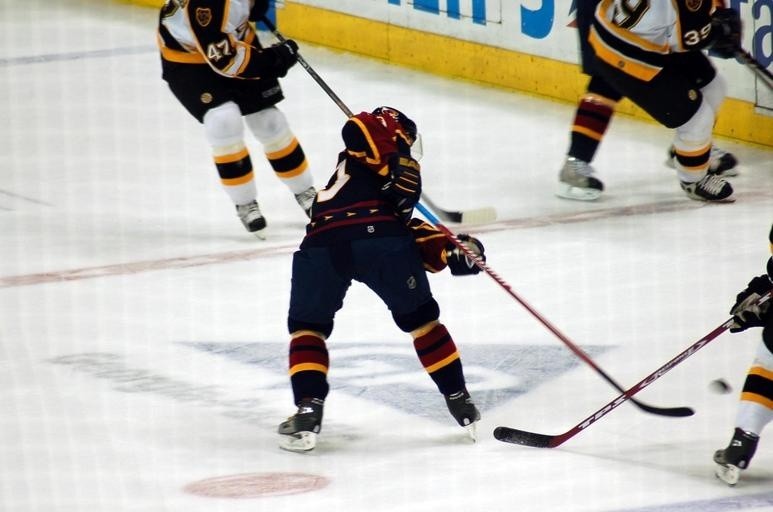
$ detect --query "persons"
[275,105,487,437]
[158,0,320,233]
[558,1,743,200]
[711,222,773,471]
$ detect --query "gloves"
[445,233,488,277]
[380,154,425,218]
[705,6,744,61]
[239,39,299,84]
[729,274,773,334]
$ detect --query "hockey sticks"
[415,205,695,417]
[494,293,770,448]
[265,18,496,225]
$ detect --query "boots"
[275,397,325,437]
[558,152,606,193]
[442,386,484,428]
[713,426,761,470]
[294,185,318,220]
[233,199,270,233]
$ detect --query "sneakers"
[678,173,736,201]
[666,143,741,174]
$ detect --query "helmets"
[370,105,419,153]
